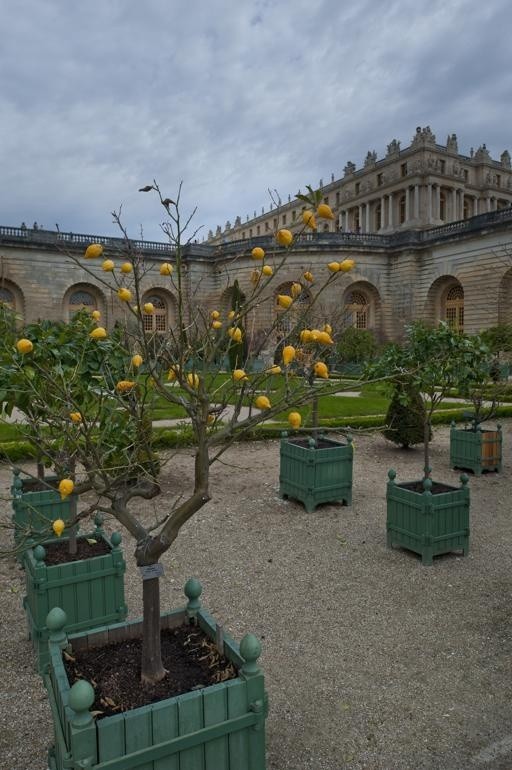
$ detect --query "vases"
[37,577,267,770]
[279,430,354,513]
[12,474,79,546]
[21,513,128,675]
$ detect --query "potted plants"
[363,316,512,566]
